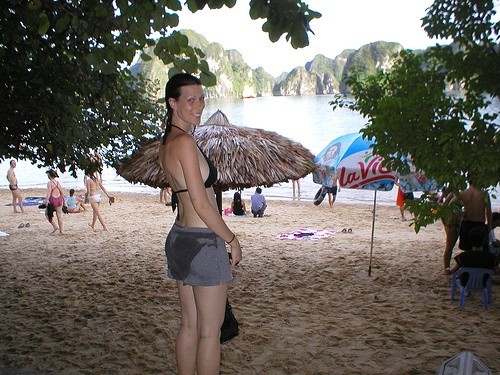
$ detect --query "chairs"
[451,268,494,310]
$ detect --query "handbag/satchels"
[220,298,240,343]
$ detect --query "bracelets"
[228,235,235,244]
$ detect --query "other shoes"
[348,228,352,232]
[340,228,347,232]
[25,223,30,227]
[17,223,24,228]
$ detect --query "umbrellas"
[313,132,445,276]
[116,110,316,218]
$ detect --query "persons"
[231,192,247,215]
[160,187,172,203]
[394,158,415,221]
[65,189,86,213]
[87,163,115,231]
[251,187,267,217]
[84,148,103,183]
[326,173,340,207]
[46,169,65,235]
[160,73,242,375]
[7,160,27,214]
[315,142,341,187]
[427,171,500,296]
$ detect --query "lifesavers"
[312,186,326,205]
